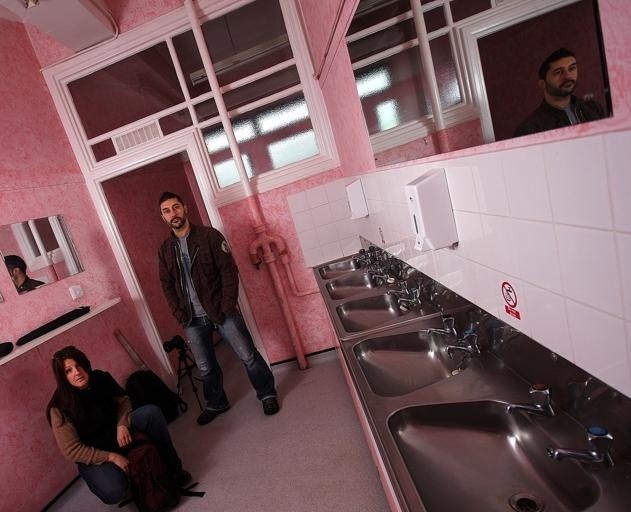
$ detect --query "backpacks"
[118,430,206,512]
[124,369,189,419]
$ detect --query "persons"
[514,49,606,137]
[158,192,280,425]
[46,346,192,505]
[4,255,46,293]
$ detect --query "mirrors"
[339,1,616,168]
[0,214,84,292]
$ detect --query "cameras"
[163,335,184,353]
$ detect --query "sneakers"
[261,396,280,416]
[195,400,231,426]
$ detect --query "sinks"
[317,257,603,511]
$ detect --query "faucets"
[350,244,487,362]
[504,382,556,422]
[547,426,619,469]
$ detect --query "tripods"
[175,350,203,411]
